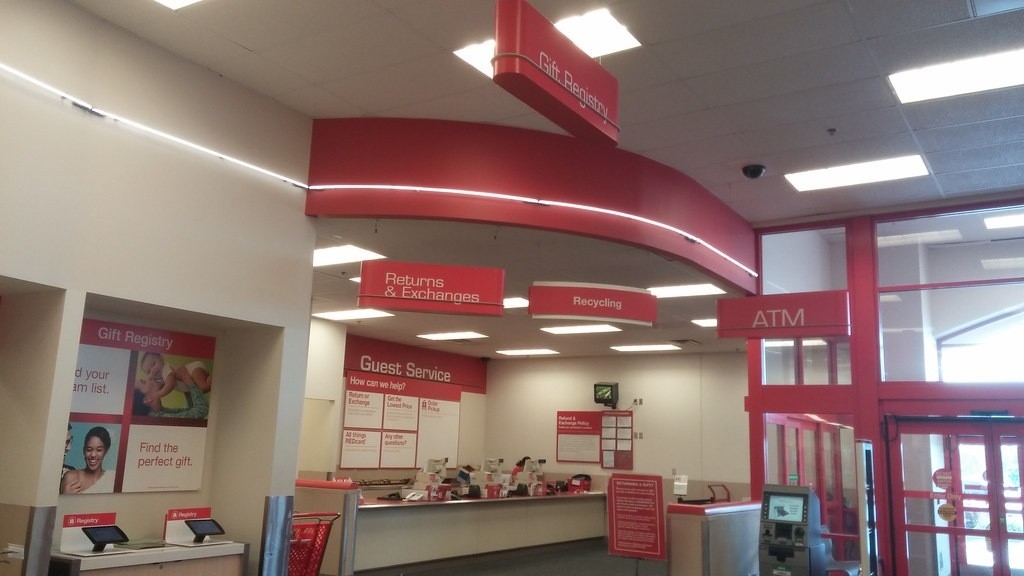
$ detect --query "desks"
[47,540,251,576]
[353,491,606,572]
[666,500,762,576]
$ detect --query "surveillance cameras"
[741,165,766,180]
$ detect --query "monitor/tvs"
[594,382,618,404]
[768,496,803,521]
[482,458,499,472]
[524,460,538,472]
[423,458,445,475]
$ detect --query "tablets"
[82,525,129,545]
[185,519,225,536]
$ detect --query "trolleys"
[289,512,341,576]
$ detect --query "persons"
[60,423,117,494]
[511,456,531,482]
[132,351,213,419]
